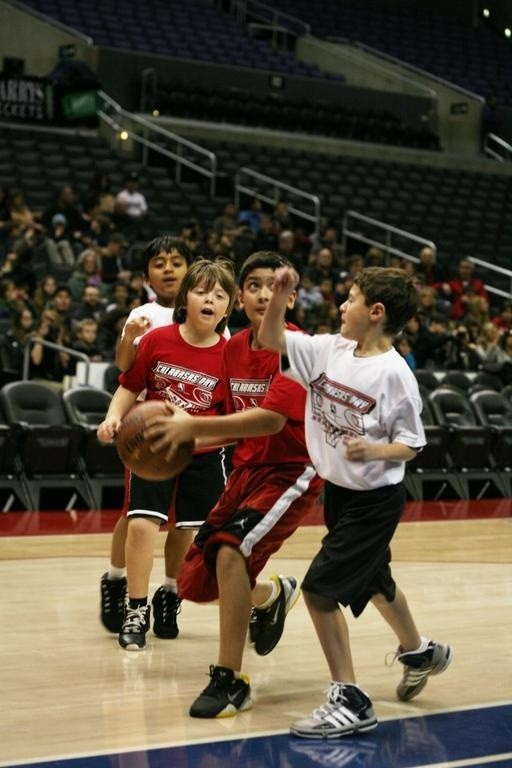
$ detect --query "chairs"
[1,382,96,513]
[63,389,131,511]
[396,365,511,501]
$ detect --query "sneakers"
[395,635,455,704]
[117,600,152,651]
[188,665,254,719]
[99,571,129,634]
[246,572,302,656]
[288,681,380,740]
[395,705,448,767]
[286,740,378,767]
[150,583,183,639]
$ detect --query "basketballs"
[114,401,196,480]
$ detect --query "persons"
[99,233,196,640]
[95,256,240,652]
[258,264,454,741]
[0,170,511,389]
[143,249,326,720]
